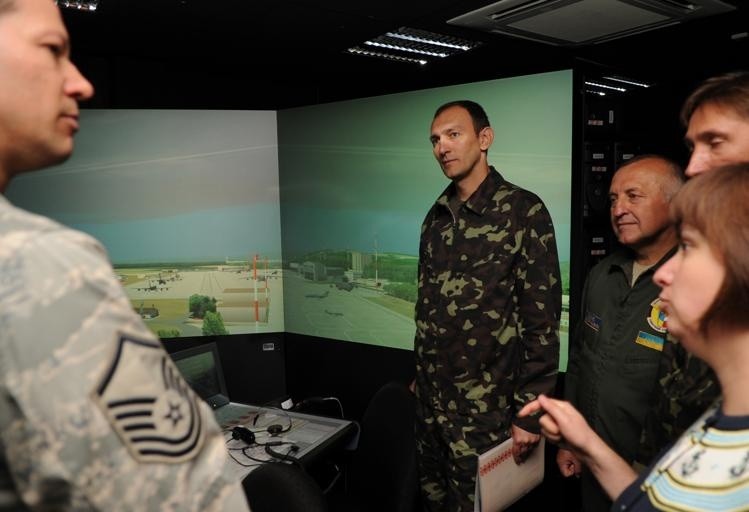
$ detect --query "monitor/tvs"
[170,342,229,408]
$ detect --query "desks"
[213,400,356,511]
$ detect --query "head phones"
[252,402,292,436]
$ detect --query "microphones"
[295,396,345,419]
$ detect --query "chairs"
[242,457,312,512]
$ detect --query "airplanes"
[130,281,173,293]
[150,271,187,288]
[215,257,251,276]
[304,291,329,300]
[323,308,343,318]
[237,271,288,282]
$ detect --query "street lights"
[374,240,379,286]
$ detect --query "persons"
[556,156,685,511]
[645,73,749,471]
[0,0,250,512]
[407,100,562,512]
[518,162,749,512]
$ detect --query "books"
[474,438,546,512]
